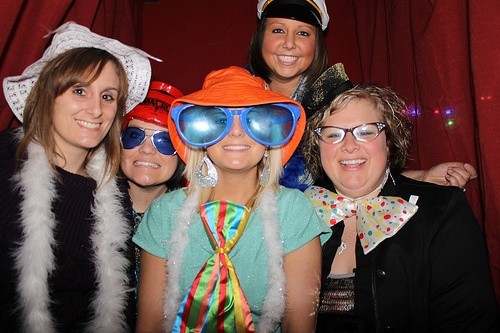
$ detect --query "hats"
[168,66,306,171]
[3,21,163,125]
[128,80,185,129]
[301,63,358,120]
[257,0,330,30]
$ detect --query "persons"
[298,62,500,333]
[117,80,187,300]
[132,66,333,333]
[0,21,152,333]
[247,0,479,188]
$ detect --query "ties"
[170,199,255,332]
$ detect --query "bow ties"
[302,186,419,255]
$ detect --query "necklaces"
[338,240,347,254]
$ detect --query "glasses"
[314,121,390,144]
[170,104,301,147]
[118,126,180,156]
